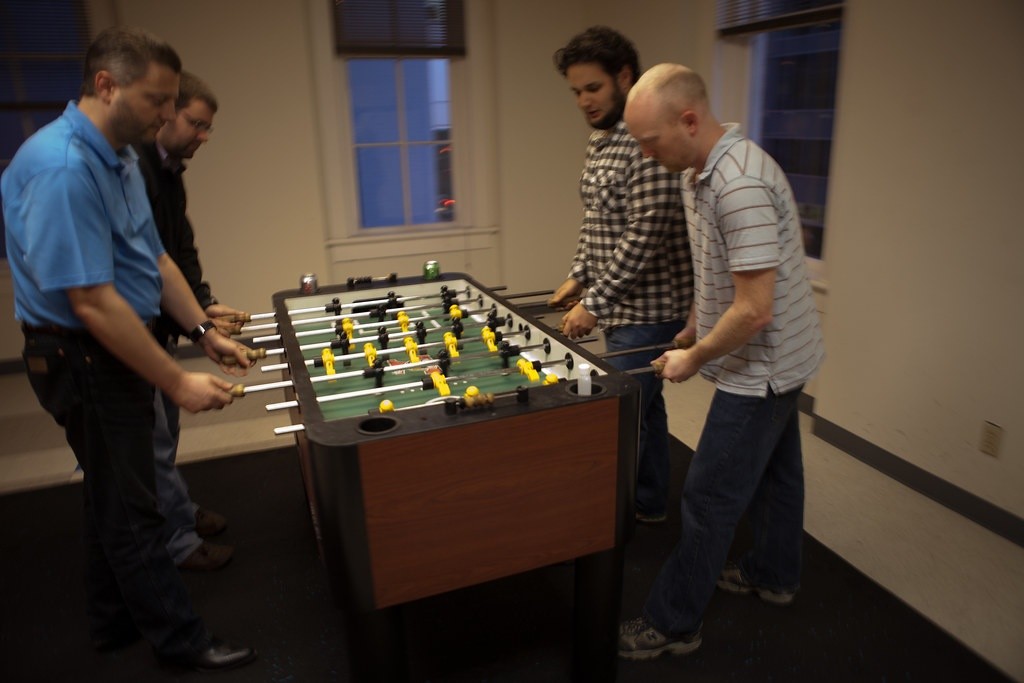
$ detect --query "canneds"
[300,273,317,290]
[423,260,439,276]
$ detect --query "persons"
[131,68,250,572]
[602,64,825,660]
[548,25,694,519]
[0,25,256,671]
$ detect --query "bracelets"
[189,320,217,343]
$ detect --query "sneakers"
[180,539,234,571]
[618,616,704,661]
[195,506,227,536]
[716,566,794,604]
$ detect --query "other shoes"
[636,513,669,522]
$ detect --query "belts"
[27,320,154,336]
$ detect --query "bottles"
[577,363,591,396]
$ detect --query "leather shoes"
[170,637,257,673]
